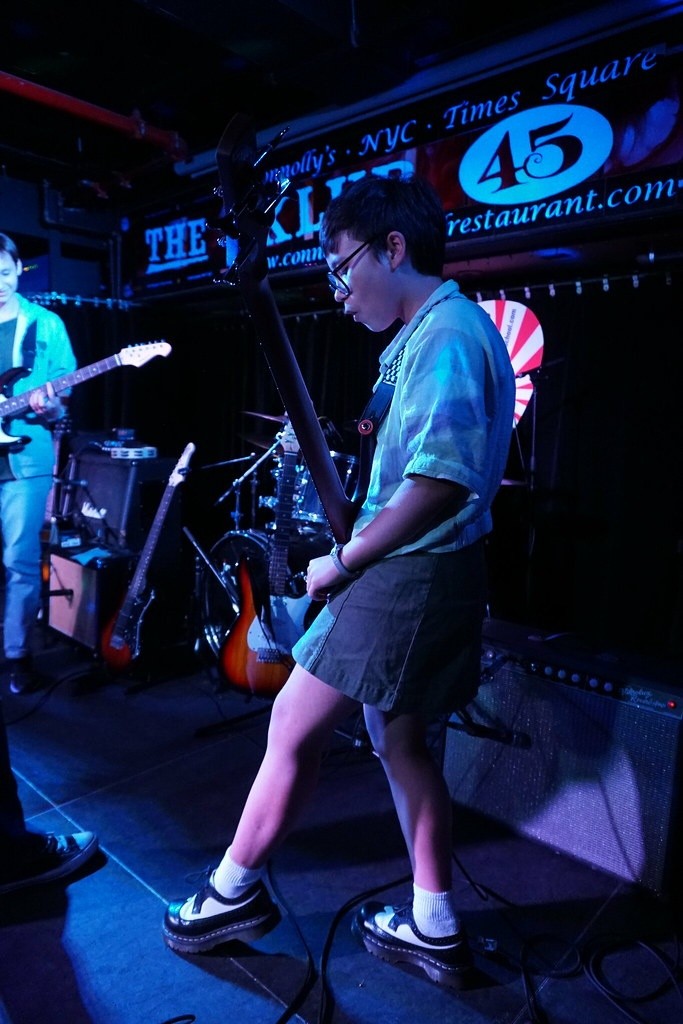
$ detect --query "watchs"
[329,544,360,580]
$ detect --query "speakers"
[439,641,683,913]
[42,443,175,659]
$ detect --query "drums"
[199,525,335,664]
[257,448,362,524]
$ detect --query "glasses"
[327,241,368,295]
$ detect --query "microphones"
[445,721,530,750]
[42,589,72,597]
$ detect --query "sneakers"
[0,831,98,893]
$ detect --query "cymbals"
[238,431,278,453]
[243,409,288,425]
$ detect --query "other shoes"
[9,657,40,694]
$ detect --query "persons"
[0,237,100,890]
[158,175,515,985]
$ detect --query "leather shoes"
[162,865,282,952]
[351,897,474,986]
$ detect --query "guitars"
[0,339,174,447]
[218,419,314,693]
[99,440,197,668]
